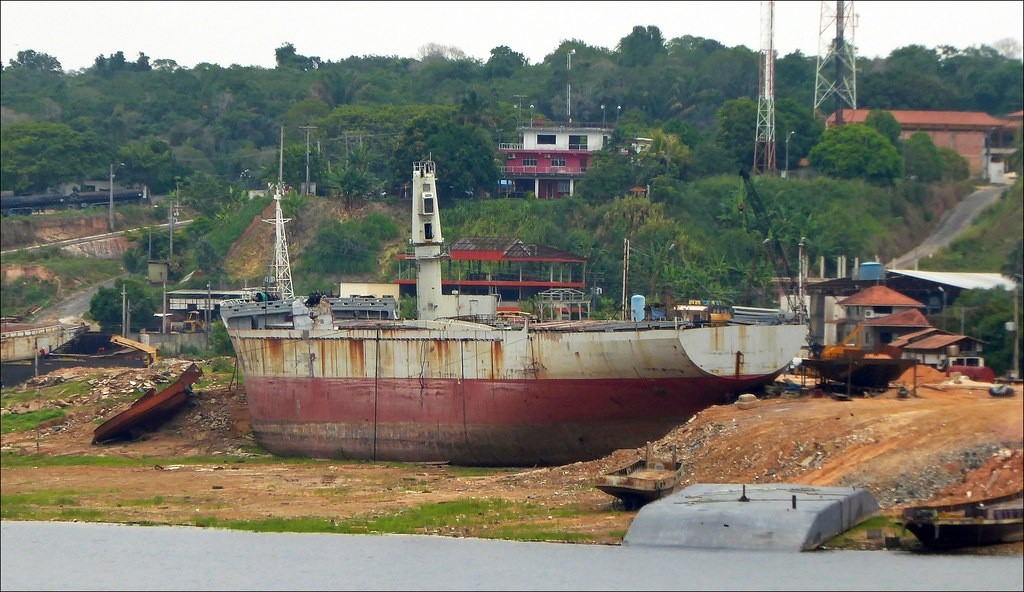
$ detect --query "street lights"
[107,162,125,231]
[786,129,796,175]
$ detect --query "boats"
[93,362,204,443]
[225,157,835,469]
[898,489,1023,551]
[593,440,684,506]
